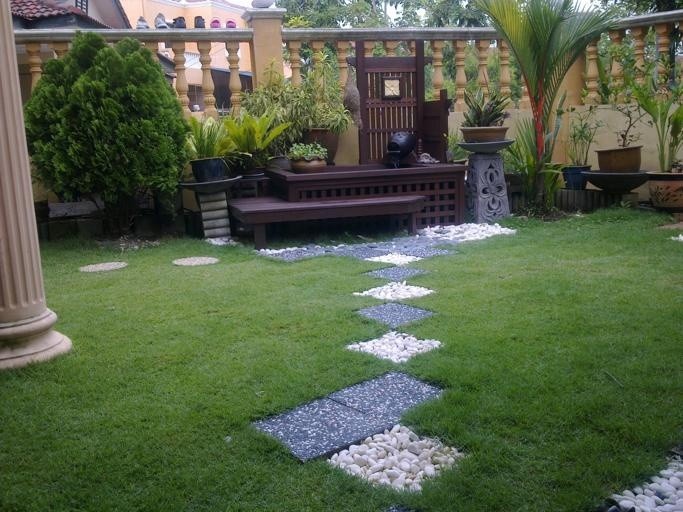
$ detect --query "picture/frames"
[380,76,402,100]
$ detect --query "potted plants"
[286,135,328,174]
[593,23,667,174]
[185,110,252,182]
[221,103,295,179]
[628,52,682,214]
[295,47,356,167]
[558,104,606,191]
[458,81,512,143]
